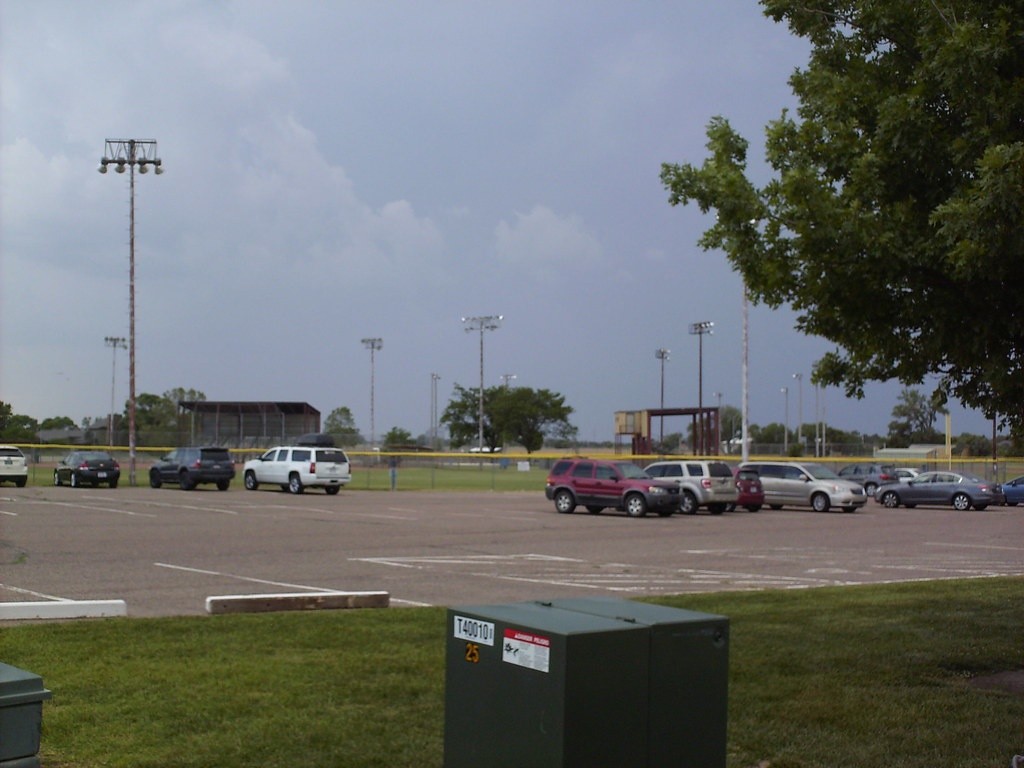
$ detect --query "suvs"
[545,457,683,518]
[242,433,352,494]
[148,446,236,491]
[894,467,923,482]
[644,461,740,515]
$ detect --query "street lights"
[690,322,714,408]
[461,314,503,471]
[793,374,803,443]
[655,348,671,460]
[103,337,128,448]
[361,336,384,465]
[780,387,788,452]
[501,374,517,386]
[96,140,165,486]
[431,373,440,452]
[714,393,725,454]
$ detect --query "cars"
[999,476,1024,506]
[0,444,29,487]
[53,450,120,488]
[874,471,1005,512]
[839,463,899,497]
[725,466,765,512]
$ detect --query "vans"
[739,461,867,513]
